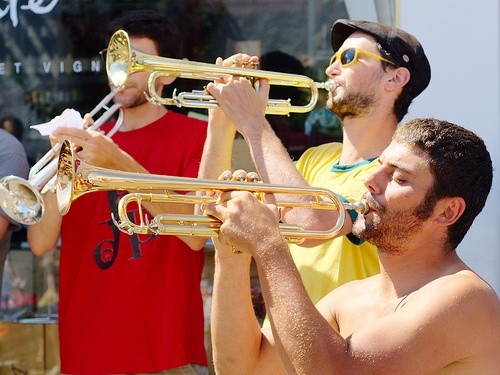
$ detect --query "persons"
[0,9,222,375]
[200,118,499,375]
[190,18,431,326]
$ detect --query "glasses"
[329,46,400,68]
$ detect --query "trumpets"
[0,85,126,225]
[56,140,367,253]
[106,30,336,115]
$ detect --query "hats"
[330,18,432,97]
